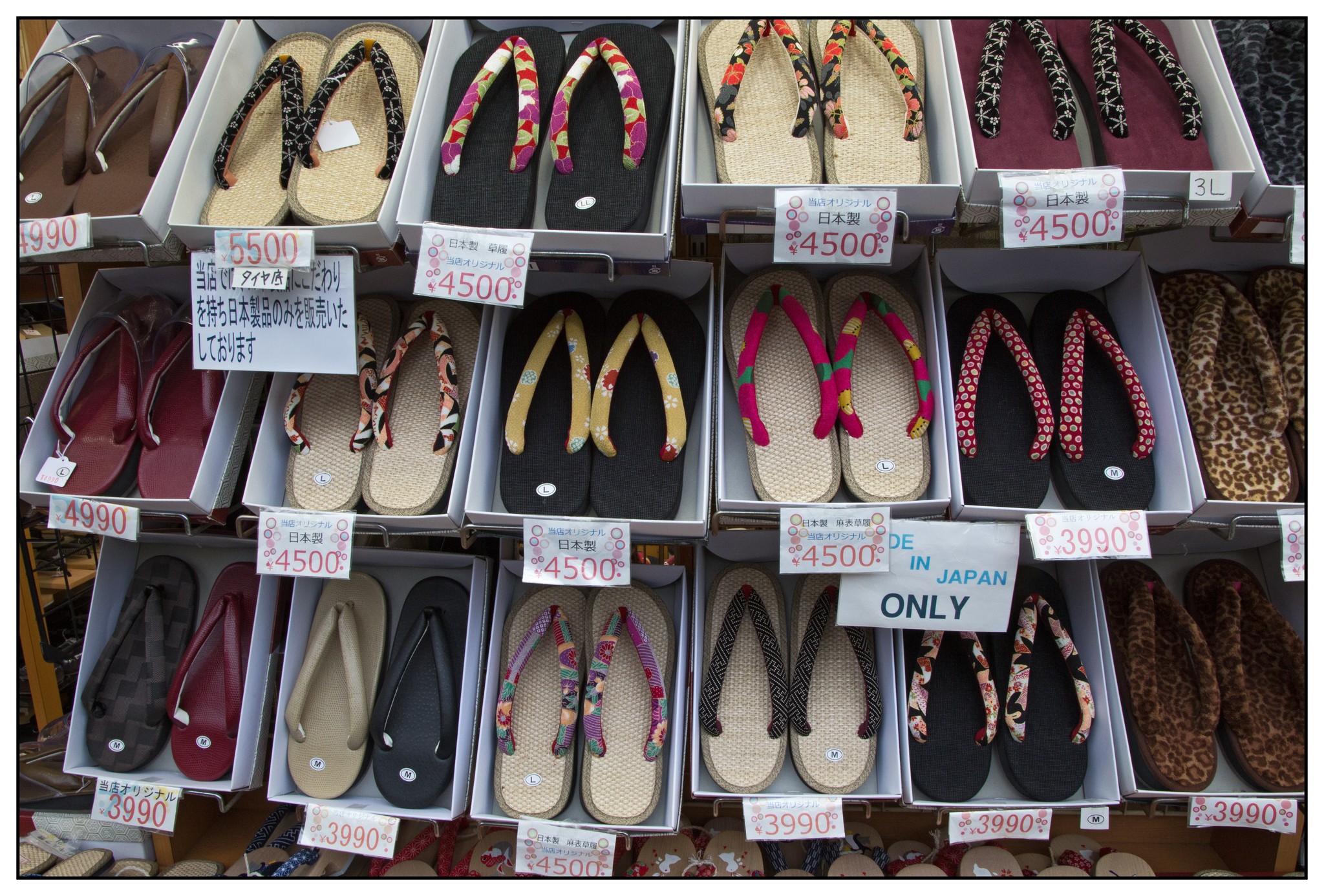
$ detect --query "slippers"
[21,14,1305,877]
